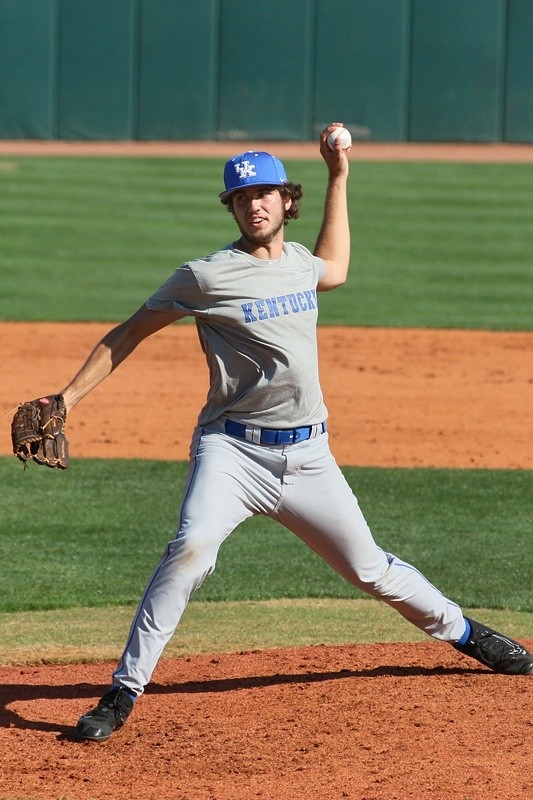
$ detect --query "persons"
[13,123,533,741]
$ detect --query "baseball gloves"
[11,393,70,469]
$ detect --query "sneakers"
[452,615,533,674]
[76,685,137,739]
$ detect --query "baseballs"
[327,126,352,150]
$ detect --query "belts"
[225,419,326,444]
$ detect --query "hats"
[219,151,289,200]
